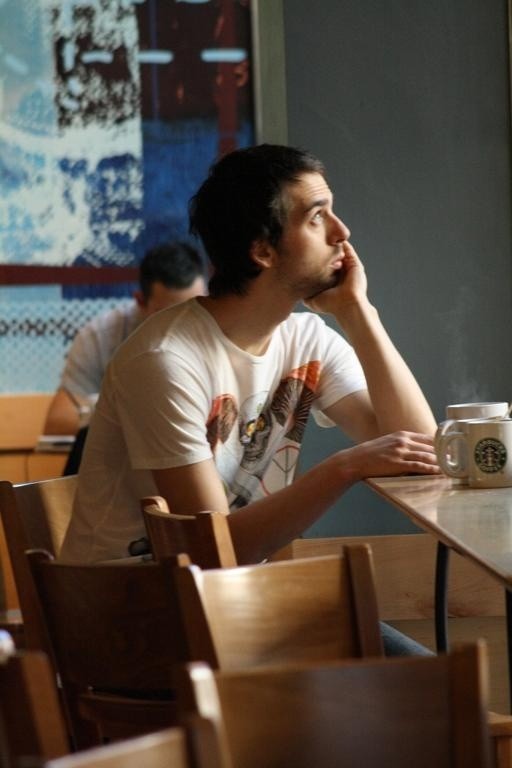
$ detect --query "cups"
[435,400,512,488]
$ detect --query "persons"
[44,242,205,436]
[57,143,444,700]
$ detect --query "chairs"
[4,641,190,768]
[0,472,165,647]
[140,493,239,571]
[181,636,494,767]
[172,541,389,671]
[26,546,196,750]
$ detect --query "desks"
[366,473,511,718]
